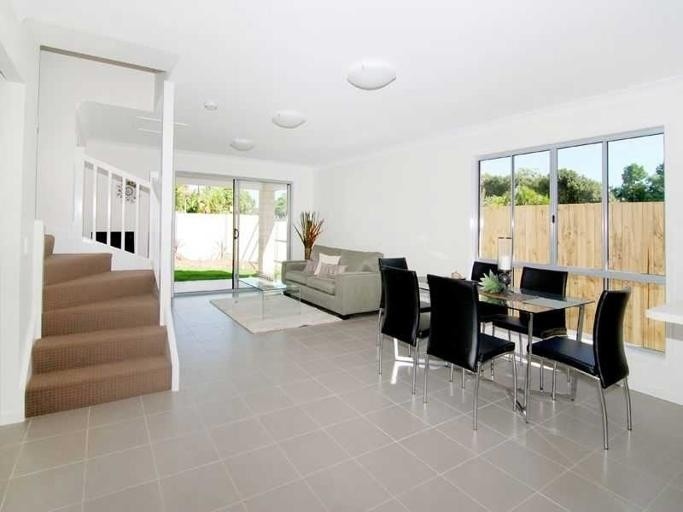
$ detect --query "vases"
[304,248,311,261]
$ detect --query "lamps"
[341,56,395,90]
[496,235,514,296]
[231,137,256,150]
[270,108,304,130]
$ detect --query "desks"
[274,259,305,265]
[405,269,594,414]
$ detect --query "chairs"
[378,263,453,396]
[374,254,431,320]
[528,288,637,453]
[489,261,570,397]
[424,271,518,431]
[472,257,514,365]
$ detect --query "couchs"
[279,244,386,319]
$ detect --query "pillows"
[314,251,341,277]
[318,262,346,279]
[302,256,316,272]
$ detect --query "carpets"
[210,293,342,335]
